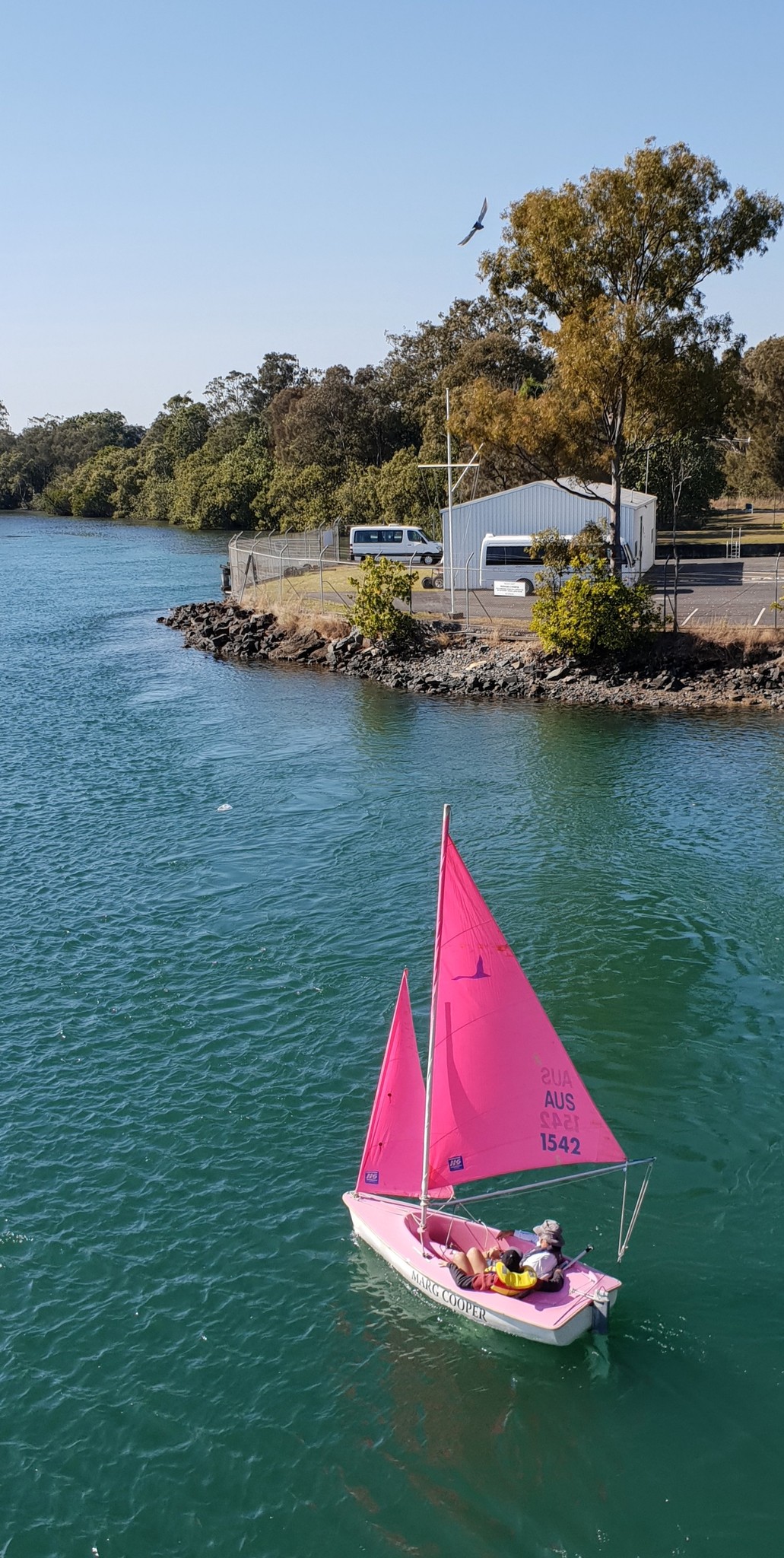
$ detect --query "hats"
[532,1219,565,1247]
[501,1246,524,1269]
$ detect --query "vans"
[478,532,638,592]
[348,523,444,565]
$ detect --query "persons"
[426,1219,566,1297]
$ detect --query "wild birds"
[458,198,489,246]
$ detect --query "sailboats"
[338,800,660,1350]
